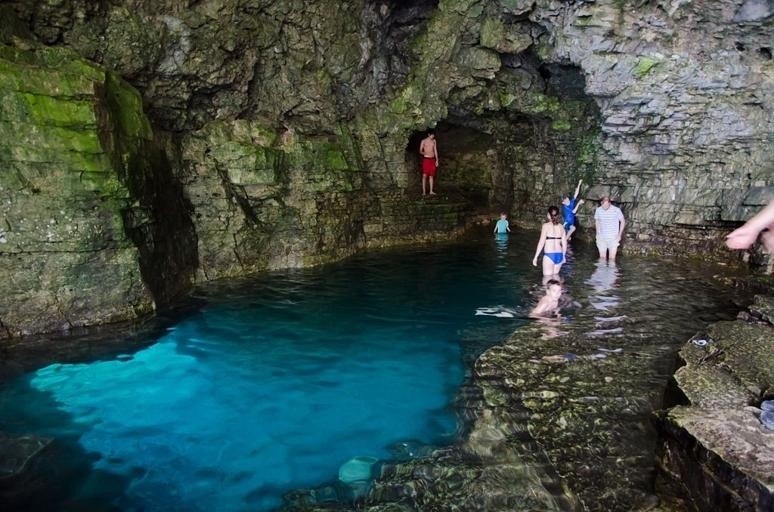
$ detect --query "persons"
[492,211,511,235]
[559,179,586,241]
[418,130,440,197]
[532,206,566,278]
[501,281,564,321]
[592,197,625,267]
[723,196,774,254]
